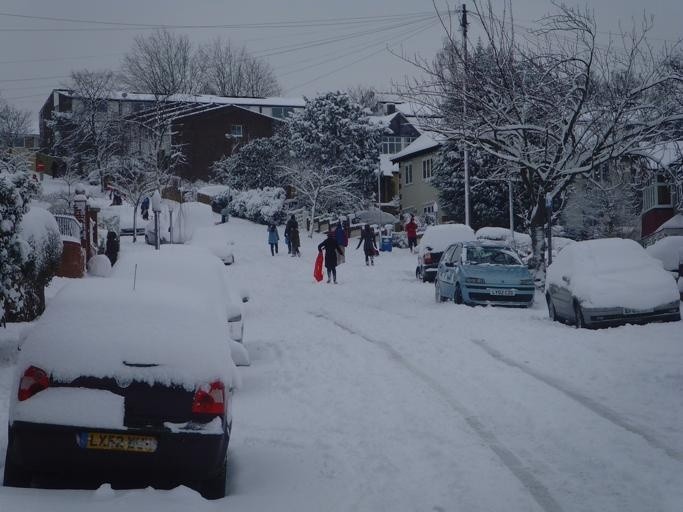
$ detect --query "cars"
[429,237,538,312]
[413,222,474,284]
[542,237,680,329]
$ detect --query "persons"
[112,191,120,204]
[405,217,417,253]
[104,232,118,266]
[268,214,301,258]
[356,223,378,266]
[313,222,349,284]
[141,196,149,220]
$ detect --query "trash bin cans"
[382,236,392,252]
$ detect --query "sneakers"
[326,280,338,284]
[337,261,345,265]
[271,250,300,259]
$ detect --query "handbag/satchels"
[368,248,380,257]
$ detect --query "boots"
[365,260,374,266]
